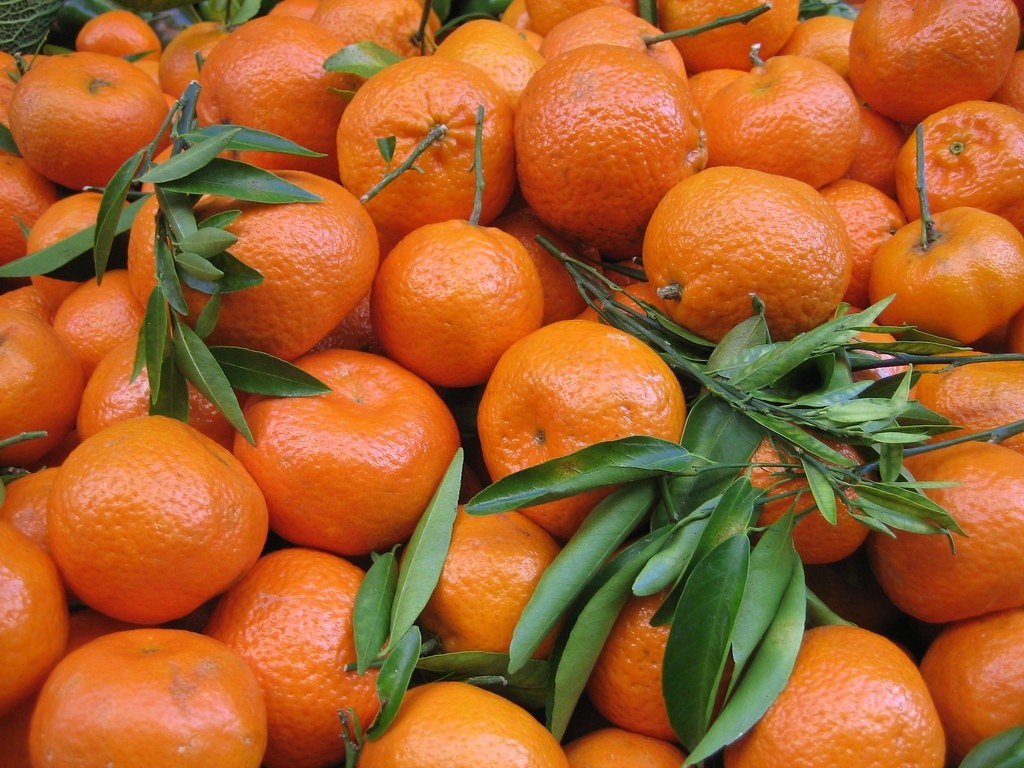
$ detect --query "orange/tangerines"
[0,0,1024,768]
